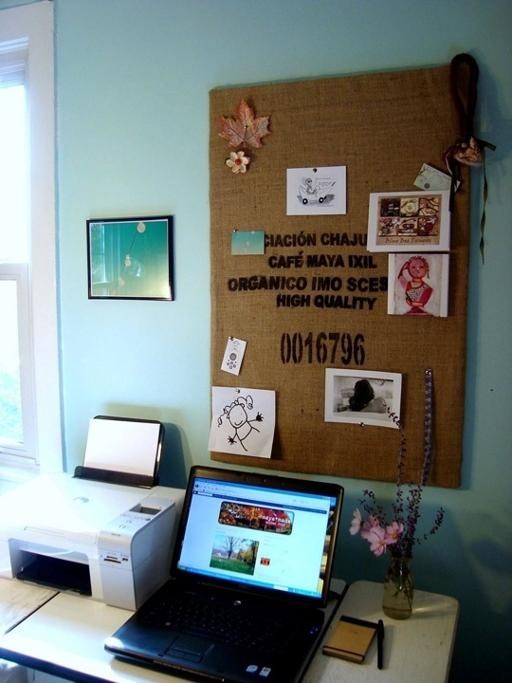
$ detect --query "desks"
[0,575,462,683]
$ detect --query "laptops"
[103,465,345,683]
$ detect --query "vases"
[383,555,414,620]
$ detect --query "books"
[322,614,381,664]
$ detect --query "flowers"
[348,399,446,611]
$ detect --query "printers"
[0,414,187,613]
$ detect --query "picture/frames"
[87,216,174,301]
